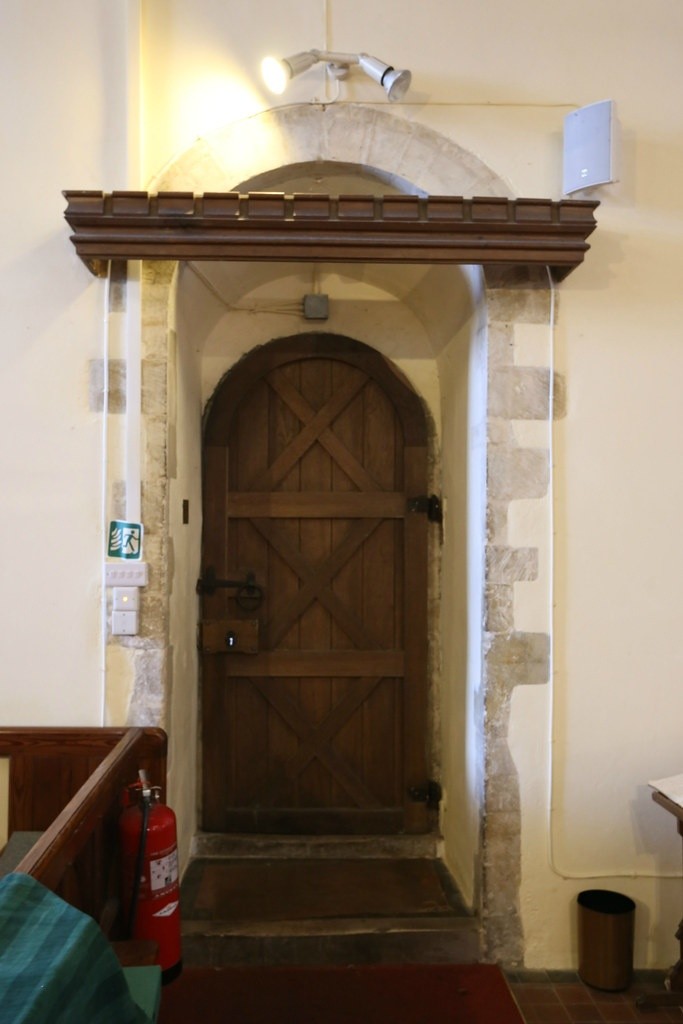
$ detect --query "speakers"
[563,97,623,196]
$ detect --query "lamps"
[258,41,417,102]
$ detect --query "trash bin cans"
[577,889,637,992]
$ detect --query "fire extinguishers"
[118,768,183,988]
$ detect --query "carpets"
[151,963,527,1024]
[177,857,477,922]
